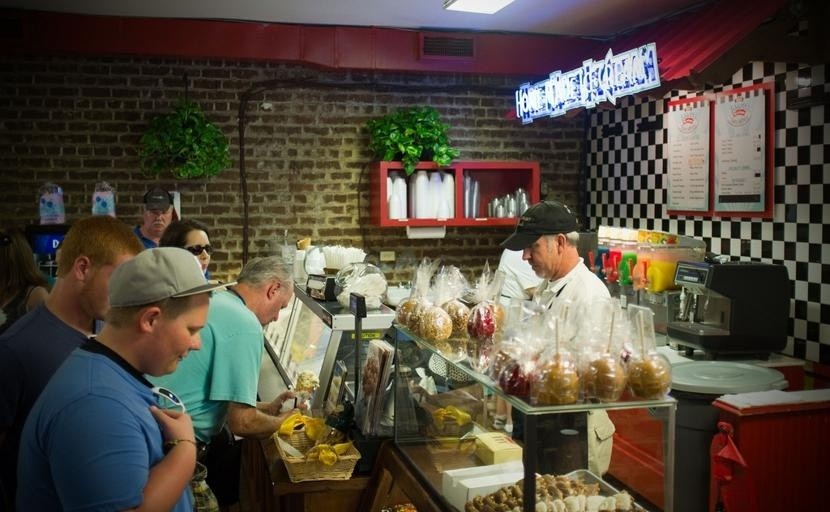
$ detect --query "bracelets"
[164,439,196,447]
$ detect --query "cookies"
[465,478,526,512]
[536,474,635,511]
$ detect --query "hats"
[108,247,237,307]
[143,189,172,211]
[499,201,578,251]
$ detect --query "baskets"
[273,426,361,483]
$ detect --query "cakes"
[362,351,380,408]
[420,307,453,343]
[489,302,505,332]
[627,359,669,398]
[444,301,471,333]
[399,301,419,324]
[583,358,626,401]
[467,306,496,339]
[538,364,579,405]
[294,370,319,407]
[407,302,430,333]
[499,361,540,396]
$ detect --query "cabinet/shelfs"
[371,323,678,512]
[369,161,541,239]
[237,278,398,512]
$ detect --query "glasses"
[0,235,12,246]
[184,245,214,255]
[148,387,186,414]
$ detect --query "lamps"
[443,0,516,14]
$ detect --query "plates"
[564,468,647,511]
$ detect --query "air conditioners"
[417,33,479,61]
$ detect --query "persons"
[499,202,612,343]
[133,187,209,283]
[494,247,546,308]
[18,248,238,512]
[0,225,51,332]
[0,214,147,512]
[144,256,308,511]
[159,219,211,274]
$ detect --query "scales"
[344,293,367,406]
